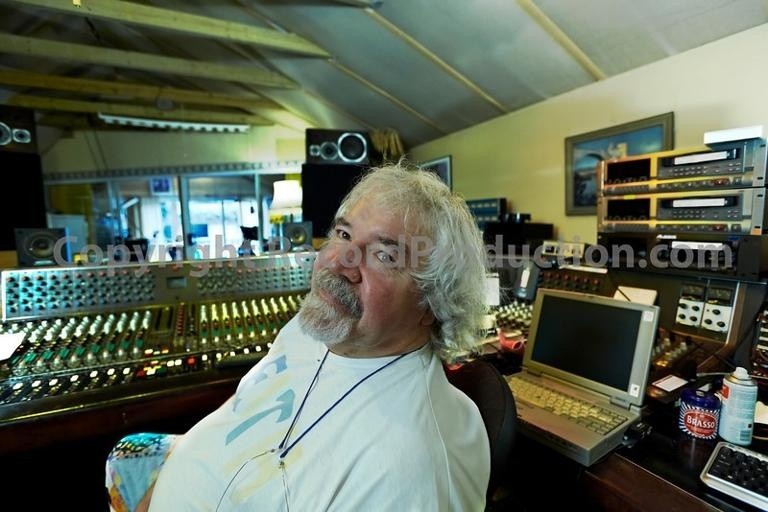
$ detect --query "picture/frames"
[563,110,673,217]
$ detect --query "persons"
[131,153,496,512]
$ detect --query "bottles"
[678,388,722,443]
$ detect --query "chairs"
[451,363,536,511]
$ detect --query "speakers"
[15,226,68,267]
[1,103,50,251]
[280,222,313,254]
[306,128,373,241]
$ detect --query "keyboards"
[701,440,768,511]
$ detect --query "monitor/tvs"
[149,176,173,196]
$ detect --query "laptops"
[502,286,661,466]
